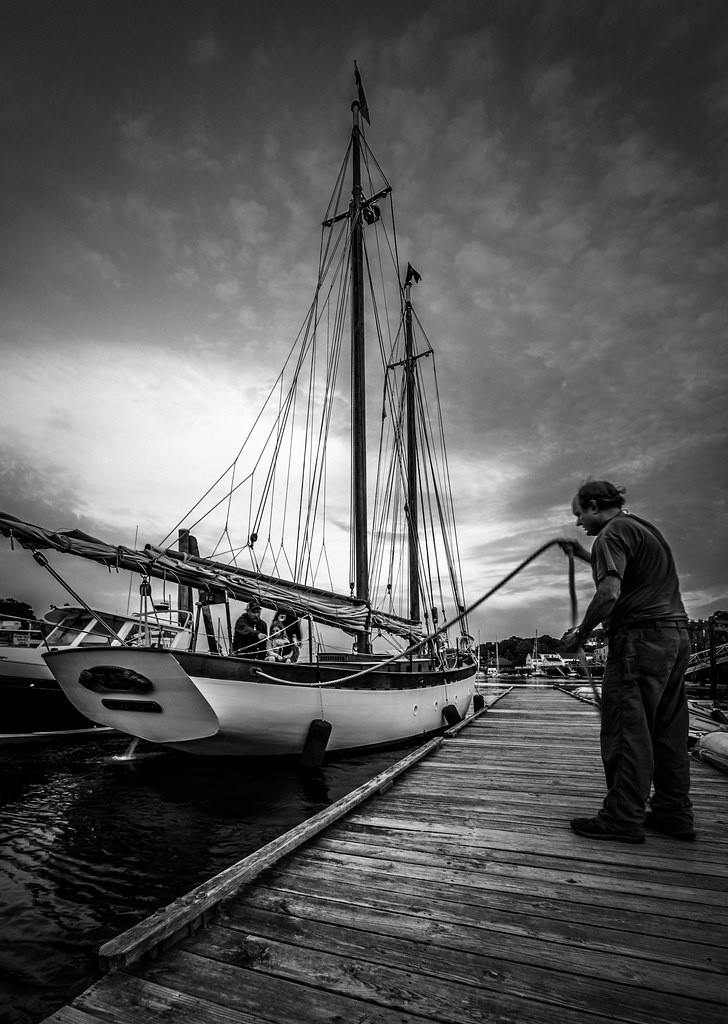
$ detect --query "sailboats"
[0,57,477,762]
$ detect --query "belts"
[619,620,687,630]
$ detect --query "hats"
[245,601,262,609]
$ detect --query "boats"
[526,649,728,773]
[0,604,194,704]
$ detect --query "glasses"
[250,610,260,614]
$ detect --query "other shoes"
[570,816,643,841]
[642,811,695,840]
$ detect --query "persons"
[234,603,303,664]
[562,481,696,844]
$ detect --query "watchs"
[573,627,586,642]
